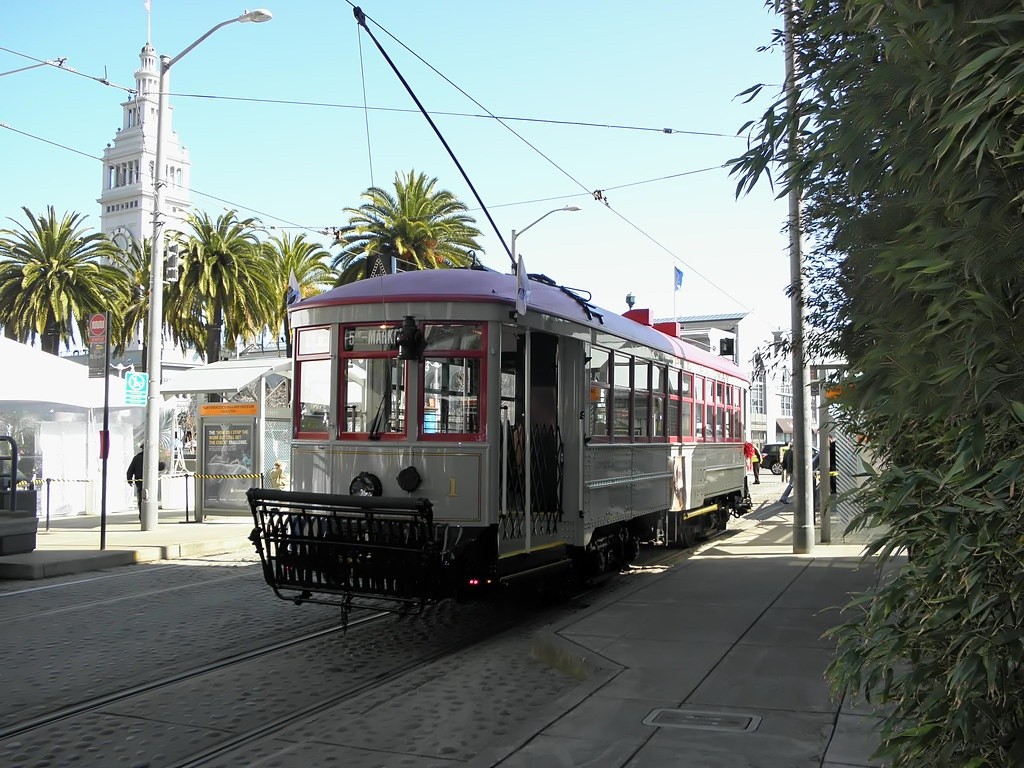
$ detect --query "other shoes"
[753,480,760,484]
[779,499,789,504]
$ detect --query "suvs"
[760,443,819,475]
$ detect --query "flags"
[287,270,302,319]
[674,267,683,292]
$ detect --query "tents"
[0,335,135,476]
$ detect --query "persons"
[270,462,286,510]
[779,441,790,483]
[779,443,794,504]
[751,442,762,484]
[126,444,144,521]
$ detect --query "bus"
[244,268,753,619]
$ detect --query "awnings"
[776,418,793,433]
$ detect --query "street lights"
[509,206,582,276]
[140,8,274,530]
